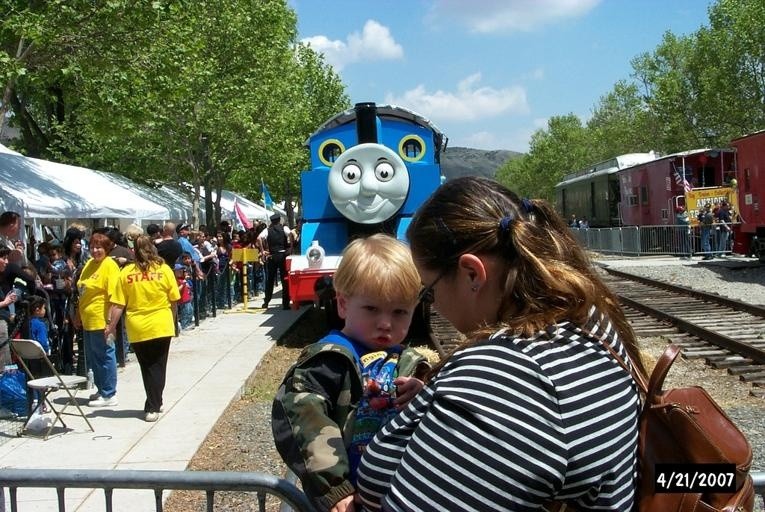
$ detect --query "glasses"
[417,275,442,304]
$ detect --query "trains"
[552,129,765,267]
[283,103,448,321]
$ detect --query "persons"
[562,201,740,259]
[356,177,657,512]
[1,213,301,420]
[270,233,436,511]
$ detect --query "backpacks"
[582,319,755,512]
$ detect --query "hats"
[176,223,190,232]
[174,263,186,270]
[270,214,281,223]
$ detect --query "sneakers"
[90,391,103,399]
[145,411,159,422]
[88,393,118,406]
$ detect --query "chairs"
[6,336,95,440]
